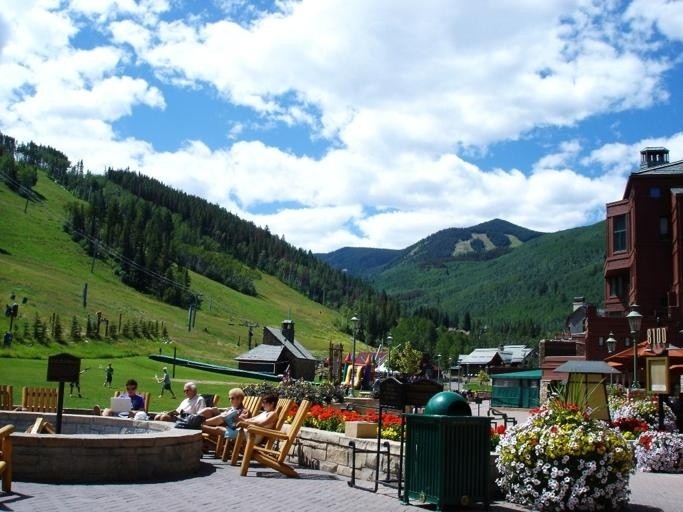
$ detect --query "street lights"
[437,355,442,381]
[387,335,393,378]
[449,358,452,391]
[626,304,643,389]
[351,317,359,397]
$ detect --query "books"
[220,410,239,431]
[110,396,131,414]
[162,408,179,417]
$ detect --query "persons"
[69,367,88,397]
[452,388,479,402]
[102,363,114,387]
[156,366,176,399]
[153,381,207,422]
[199,391,278,446]
[92,379,145,419]
[180,386,250,427]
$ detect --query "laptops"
[111,398,131,413]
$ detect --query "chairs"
[200,394,310,477]
[115,390,149,413]
[487,407,517,428]
[22,386,57,413]
[0,424,15,491]
[0,384,13,410]
